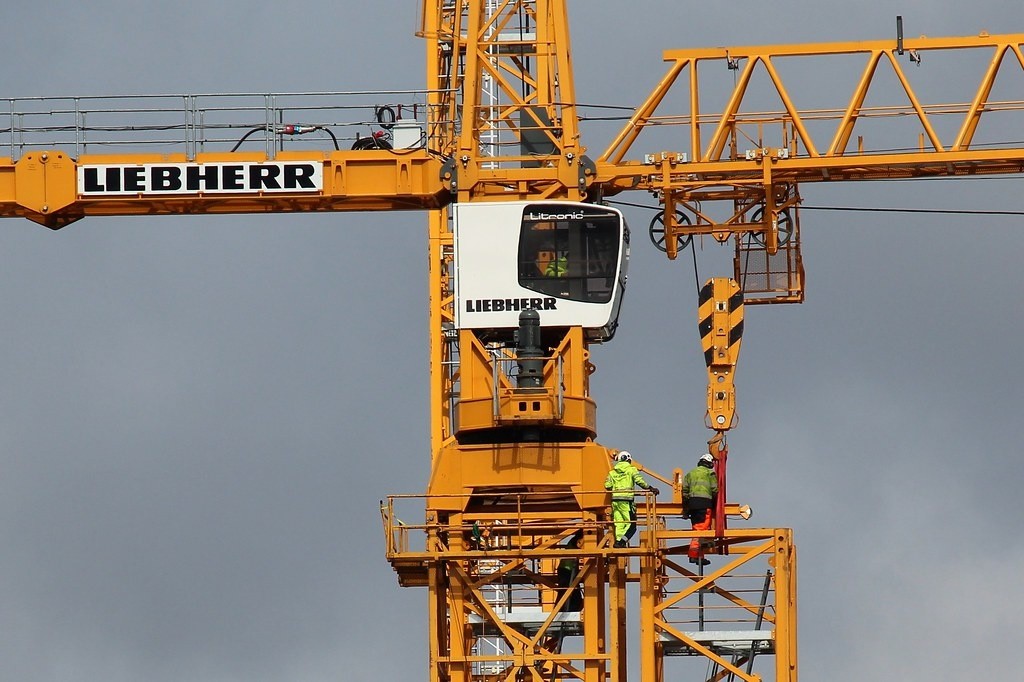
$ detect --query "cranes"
[0,0,1024,682]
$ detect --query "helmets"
[700,454,713,462]
[618,451,632,462]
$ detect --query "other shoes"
[614,542,627,548]
[690,557,710,565]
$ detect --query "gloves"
[683,510,688,518]
[649,486,659,494]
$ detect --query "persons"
[554,531,581,610]
[683,454,718,565]
[605,450,659,548]
[545,253,568,278]
